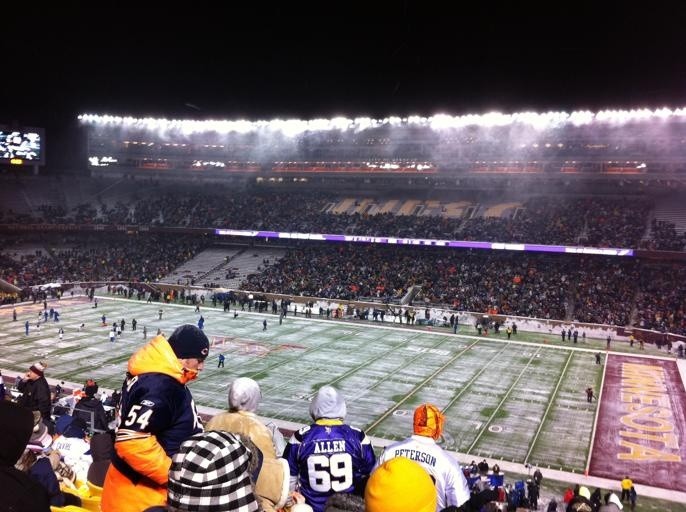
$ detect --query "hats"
[363,455,437,512]
[228,377,262,413]
[85,385,95,396]
[167,324,210,361]
[28,361,47,377]
[413,403,443,441]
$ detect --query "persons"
[1,109,686,512]
